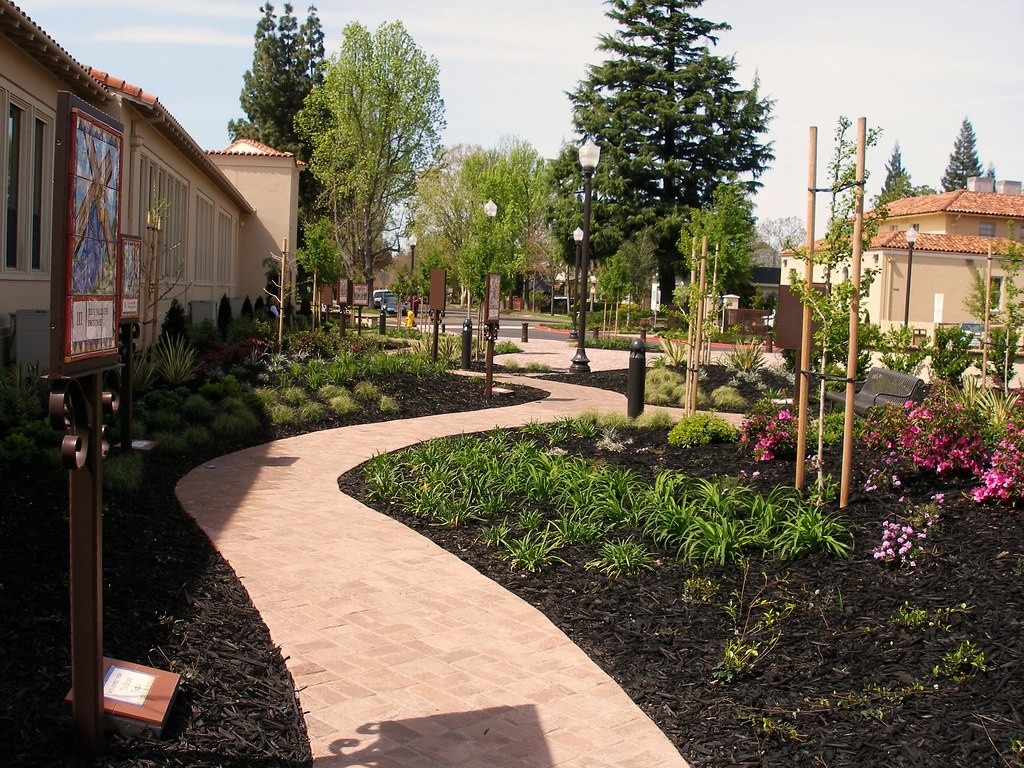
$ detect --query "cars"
[372,290,391,310]
[382,295,407,315]
[547,298,576,311]
[958,321,987,348]
[764,310,775,329]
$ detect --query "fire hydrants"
[406,311,415,327]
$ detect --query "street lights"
[570,135,613,373]
[407,233,417,327]
[902,226,918,336]
[571,227,584,340]
[486,199,499,339]
